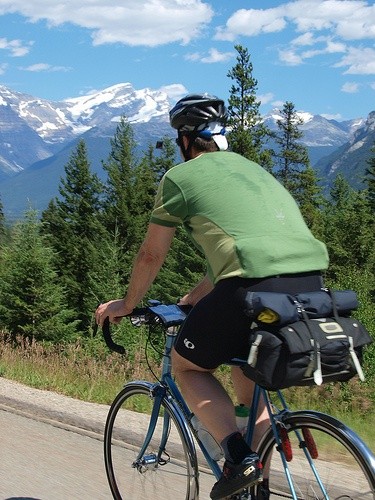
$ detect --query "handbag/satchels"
[242,289,372,390]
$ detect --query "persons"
[97,94,330,500]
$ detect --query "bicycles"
[104,306,374,500]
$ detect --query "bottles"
[188,412,224,461]
[234,404,249,436]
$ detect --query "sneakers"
[210,453,263,500]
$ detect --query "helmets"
[169,93,228,128]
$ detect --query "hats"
[180,121,226,139]
